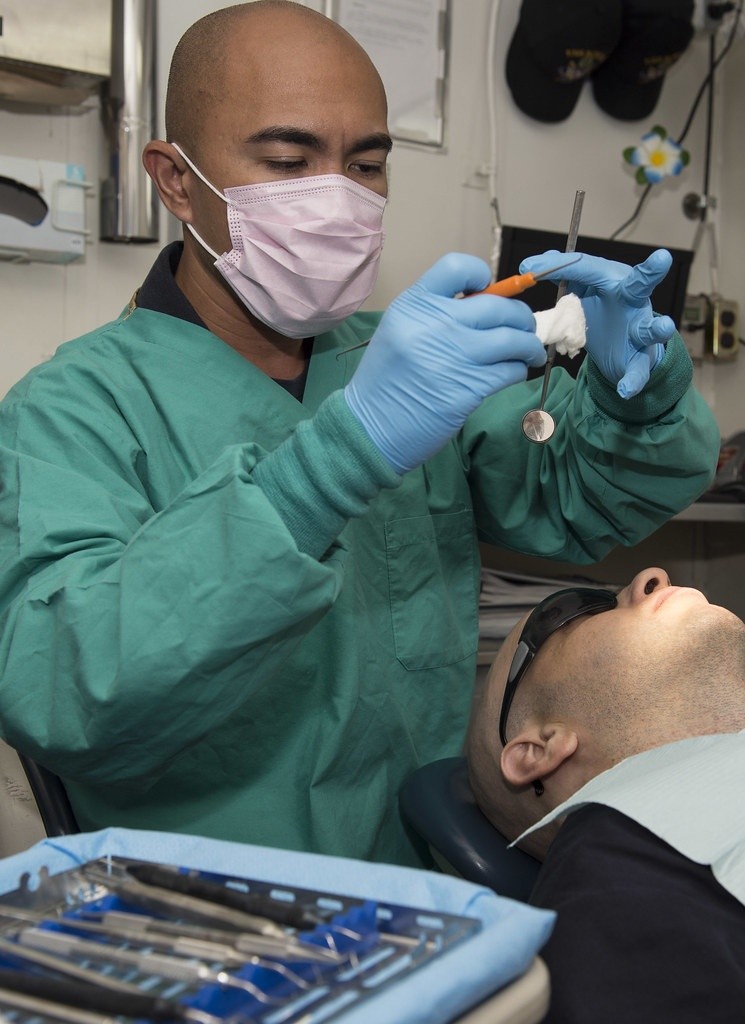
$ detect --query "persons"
[2,0,721,871]
[470,567,745,1024]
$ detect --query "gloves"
[344,252,548,477]
[518,249,678,400]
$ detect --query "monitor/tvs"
[491,224,696,383]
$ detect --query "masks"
[173,142,387,339]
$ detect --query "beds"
[397,758,540,907]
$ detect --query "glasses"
[499,587,617,797]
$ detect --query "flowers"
[621,124,691,186]
